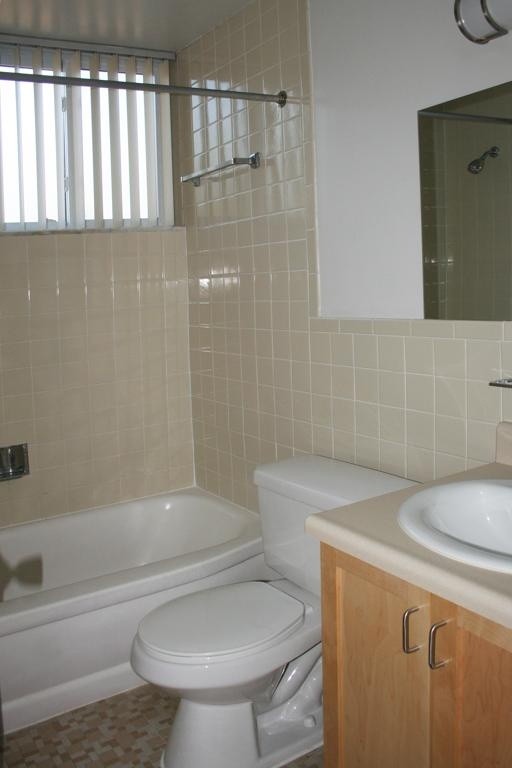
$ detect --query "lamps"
[454,0,512,44]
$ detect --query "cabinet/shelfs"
[321,541,512,768]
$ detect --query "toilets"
[129,453,424,768]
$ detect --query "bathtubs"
[0,485,287,736]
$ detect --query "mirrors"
[418,81,512,321]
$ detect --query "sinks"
[395,479,512,575]
